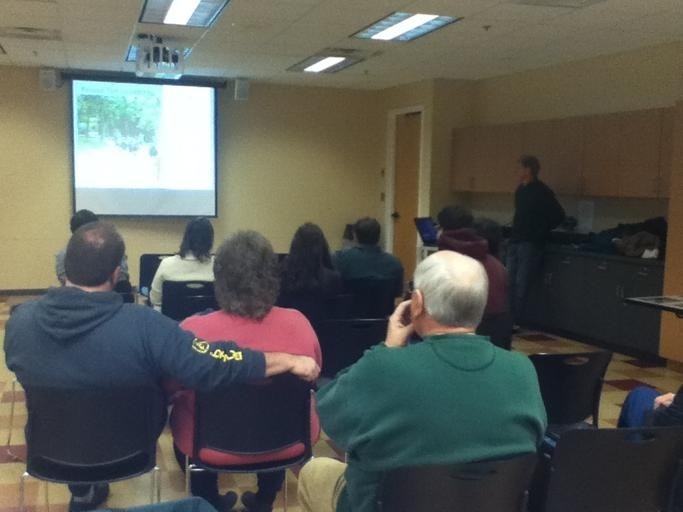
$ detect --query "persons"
[613,380,683,429]
[145,216,217,318]
[287,246,554,512]
[0,217,325,512]
[272,220,343,296]
[55,206,132,291]
[328,213,406,309]
[164,225,326,512]
[502,151,567,334]
[429,204,519,317]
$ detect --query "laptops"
[413,217,441,247]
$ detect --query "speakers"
[234,79,250,101]
[39,68,56,92]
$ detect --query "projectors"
[135,44,182,80]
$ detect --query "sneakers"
[217,491,238,511]
[242,492,273,512]
[69,481,110,512]
